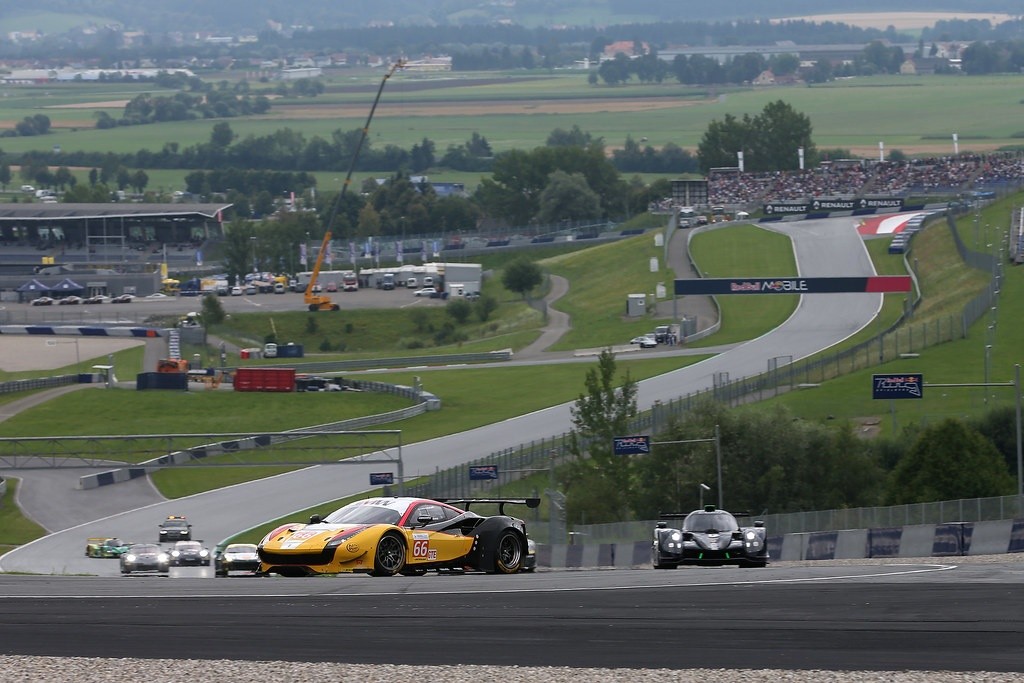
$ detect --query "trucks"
[382,274,396,290]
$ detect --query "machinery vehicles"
[303,50,408,311]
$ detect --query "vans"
[408,278,418,288]
[423,277,434,286]
[654,325,669,340]
[274,283,286,293]
[232,287,243,295]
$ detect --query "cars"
[60,295,81,305]
[630,336,657,347]
[120,543,170,573]
[164,539,210,566]
[32,296,53,305]
[83,297,103,304]
[215,544,261,576]
[112,294,132,303]
[158,516,193,541]
[86,537,129,558]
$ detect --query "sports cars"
[255,494,541,575]
[651,503,769,569]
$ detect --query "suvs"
[414,287,436,297]
